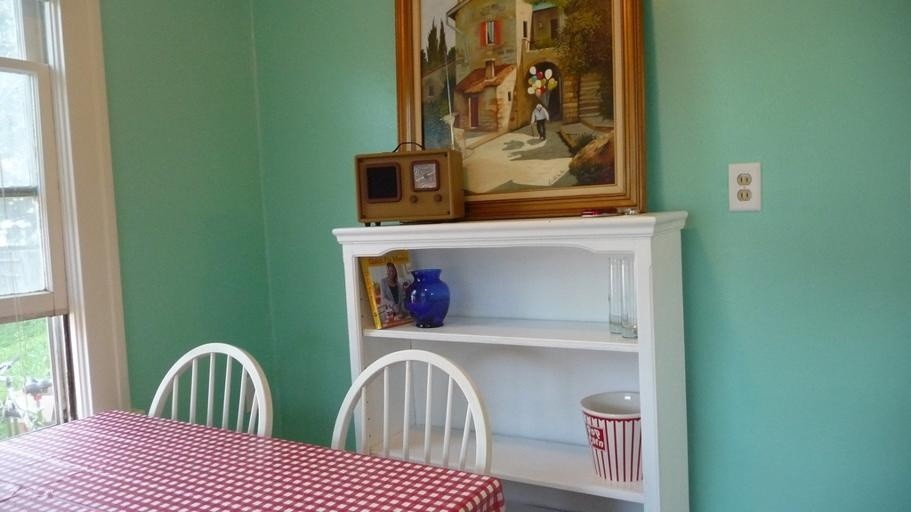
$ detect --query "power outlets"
[728,162,762,212]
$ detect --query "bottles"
[608,255,639,338]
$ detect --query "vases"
[577,390,641,485]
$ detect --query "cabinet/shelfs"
[331,205,691,512]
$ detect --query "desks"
[0,408,502,512]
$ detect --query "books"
[359,250,416,330]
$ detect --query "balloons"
[526,66,558,96]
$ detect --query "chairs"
[329,347,492,476]
[147,341,272,441]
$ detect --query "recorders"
[355,150,466,227]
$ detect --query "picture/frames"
[396,1,645,216]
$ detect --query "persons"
[379,262,411,318]
[530,104,549,141]
